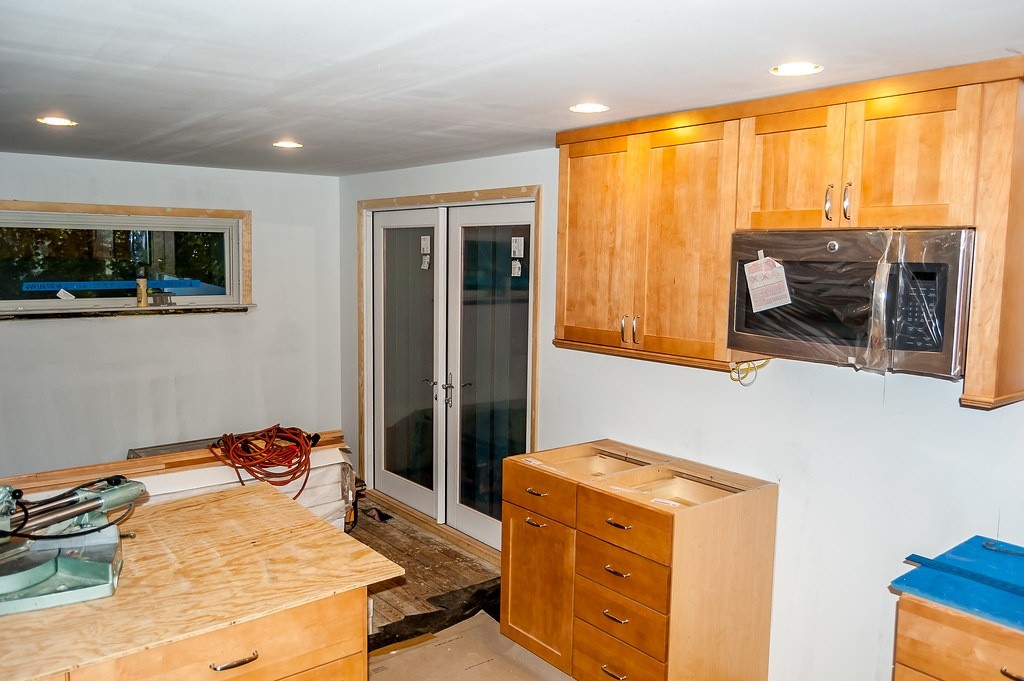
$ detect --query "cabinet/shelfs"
[496,438,780,681]
[891,535,1024,681]
[546,100,779,373]
[733,54,1024,411]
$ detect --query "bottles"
[136,271,148,307]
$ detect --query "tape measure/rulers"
[905,552,1024,596]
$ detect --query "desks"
[0,480,406,681]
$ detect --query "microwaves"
[727,229,975,378]
